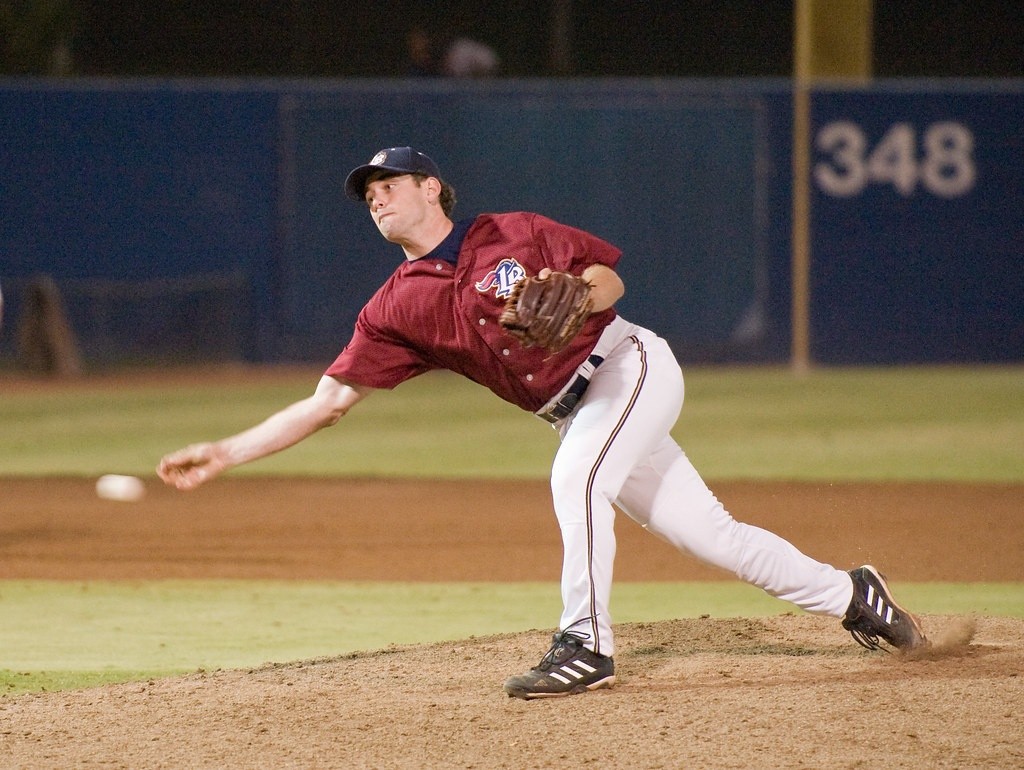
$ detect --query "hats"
[344,145,439,202]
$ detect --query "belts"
[536,355,605,423]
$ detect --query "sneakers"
[842,565,926,656]
[505,632,617,699]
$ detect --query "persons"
[409,29,496,78]
[24,33,89,79]
[156,147,929,699]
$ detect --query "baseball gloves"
[498,269,595,352]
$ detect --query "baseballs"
[98,474,144,501]
